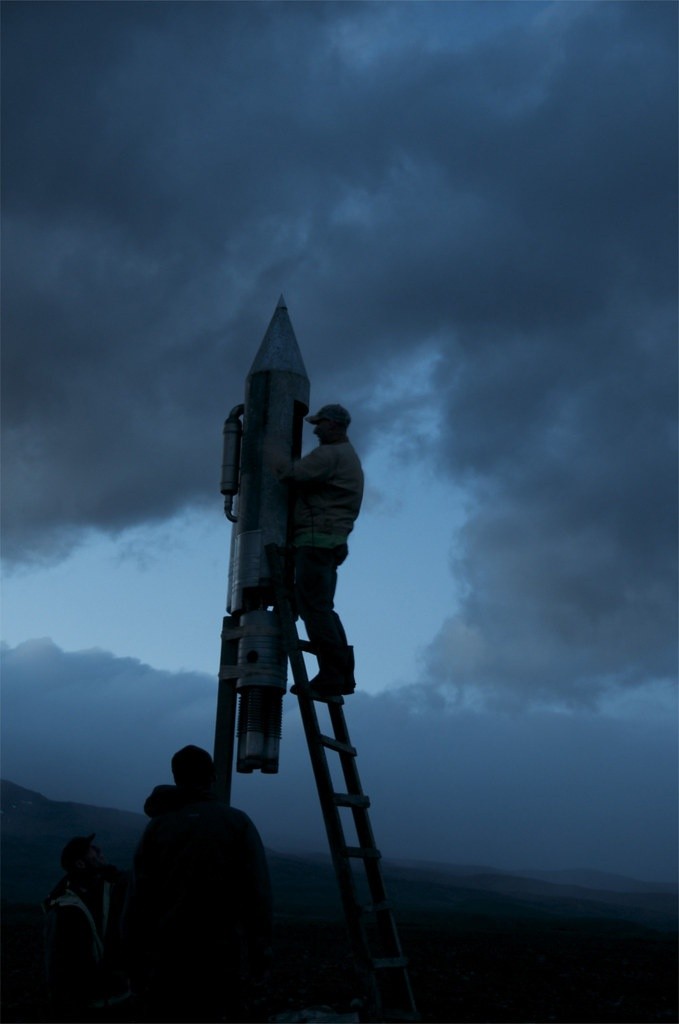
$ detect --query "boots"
[290,645,356,695]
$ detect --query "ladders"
[264,542,415,1024]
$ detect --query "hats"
[172,745,212,791]
[305,403,351,428]
[61,832,96,875]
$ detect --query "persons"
[269,403,364,697]
[40,833,130,1024]
[123,741,273,1024]
